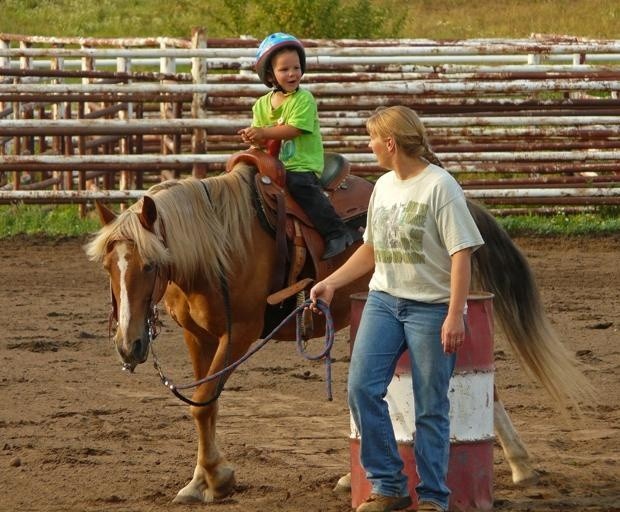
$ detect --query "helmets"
[256,32,304,88]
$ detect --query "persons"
[309,103,485,511]
[233,33,355,262]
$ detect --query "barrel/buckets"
[348,291,496,508]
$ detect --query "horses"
[80,163,598,505]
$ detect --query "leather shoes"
[319,231,355,261]
[416,502,445,512]
[355,494,413,511]
